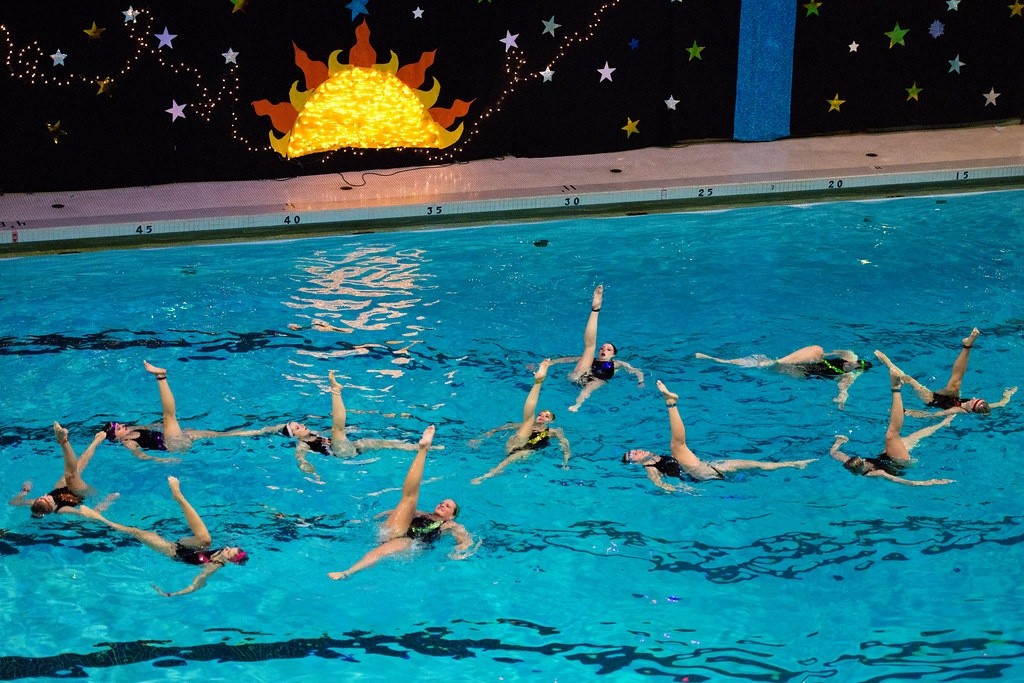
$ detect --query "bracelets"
[22,487,30,492]
[167,593,171,597]
[638,381,644,385]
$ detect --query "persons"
[828,368,961,486]
[694,345,872,411]
[9,421,119,520]
[522,285,645,412]
[79,474,250,598]
[873,326,1019,419]
[281,371,446,481]
[622,379,821,494]
[103,360,288,463]
[465,357,570,487]
[327,421,472,584]
